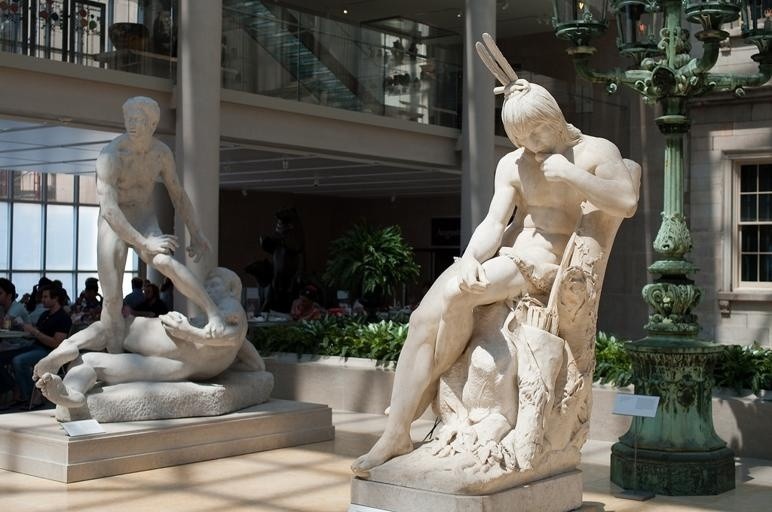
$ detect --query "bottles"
[2,314,12,330]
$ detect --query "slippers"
[21,402,44,410]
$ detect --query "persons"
[93,93,214,355]
[29,264,268,407]
[348,69,639,475]
[1,278,103,411]
[122,278,174,318]
[291,285,321,321]
